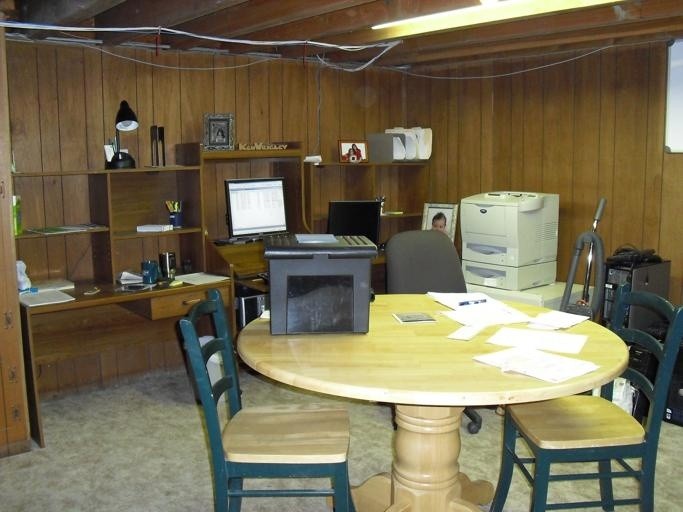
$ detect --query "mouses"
[380,243,385,251]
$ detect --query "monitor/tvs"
[224,176,288,244]
[329,200,381,244]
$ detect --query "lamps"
[110,100,140,168]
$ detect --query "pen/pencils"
[458,299,488,307]
[164,198,181,213]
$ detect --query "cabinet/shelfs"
[173,140,311,361]
[12,164,236,449]
[304,160,430,264]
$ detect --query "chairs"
[384,229,483,433]
[487,283,683,512]
[178,286,351,512]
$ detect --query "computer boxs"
[599,256,671,331]
[235,285,266,332]
[664,340,683,426]
[601,339,663,426]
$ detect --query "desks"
[236,292,631,512]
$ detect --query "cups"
[141,260,158,284]
[158,251,178,281]
[170,211,182,229]
[12,195,22,235]
[380,202,386,214]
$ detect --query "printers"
[460,188,561,291]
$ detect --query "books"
[20,290,75,307]
[31,278,75,292]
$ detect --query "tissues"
[15,258,33,292]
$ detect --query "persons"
[215,128,226,143]
[432,212,446,232]
[342,144,361,160]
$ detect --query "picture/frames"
[202,113,235,152]
[337,139,370,164]
[421,202,460,246]
